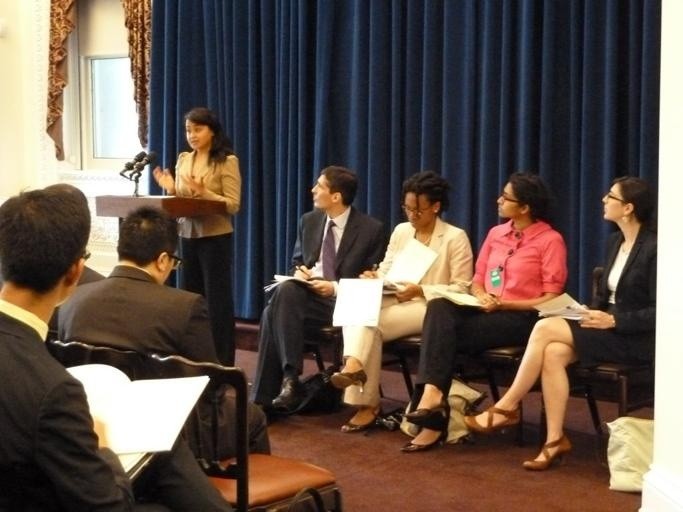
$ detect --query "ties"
[322,220,336,281]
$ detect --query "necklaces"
[621,244,632,254]
[416,232,431,244]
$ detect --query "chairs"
[379,332,423,401]
[469,344,547,443]
[565,265,654,434]
[47,339,142,380]
[297,324,343,375]
[146,353,342,512]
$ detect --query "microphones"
[129,152,156,182]
[119,151,147,175]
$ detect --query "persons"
[152,108,242,367]
[0,265,107,333]
[401,168,568,452]
[57,204,271,465]
[249,164,387,426]
[464,177,657,471]
[0,183,238,511]
[330,170,474,432]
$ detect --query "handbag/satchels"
[375,406,406,431]
[399,374,488,445]
[274,374,341,415]
[605,415,654,492]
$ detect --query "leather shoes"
[271,377,300,408]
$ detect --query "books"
[114,452,157,480]
[431,289,484,306]
[65,363,211,455]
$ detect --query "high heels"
[399,427,447,452]
[330,369,366,393]
[522,433,572,470]
[339,405,384,432]
[405,400,449,422]
[463,405,520,436]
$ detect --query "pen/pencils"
[371,263,378,271]
[296,266,311,278]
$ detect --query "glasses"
[399,204,431,216]
[168,254,184,272]
[499,193,521,204]
[83,249,90,259]
[605,194,626,204]
